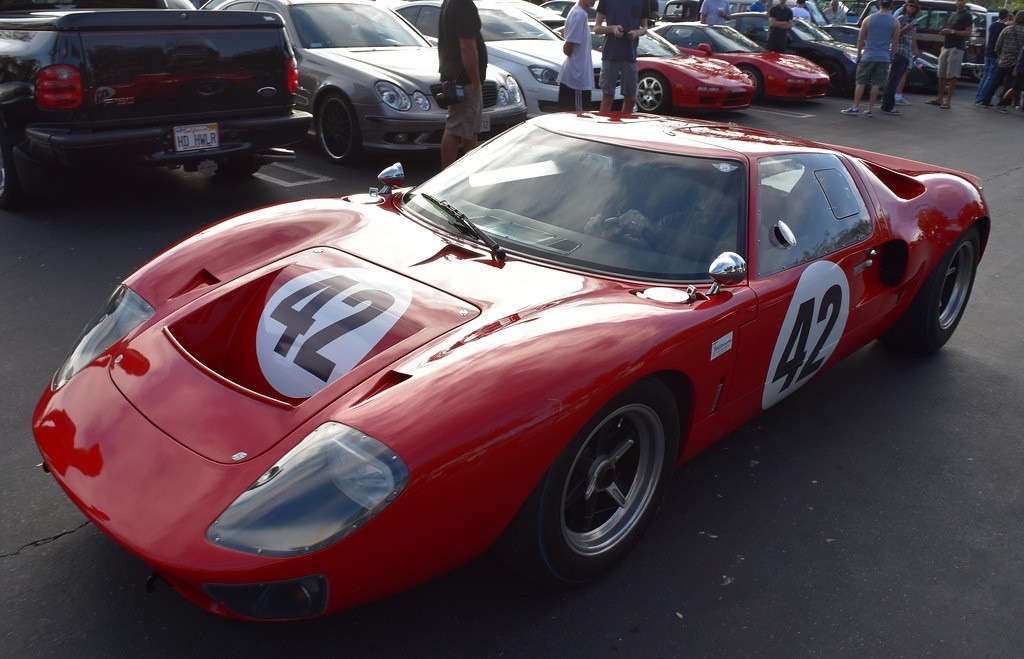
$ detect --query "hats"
[906,0,919,6]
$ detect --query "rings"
[631,221,637,226]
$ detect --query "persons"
[881,0,921,114]
[557,0,596,112]
[700,0,731,25]
[584,165,740,264]
[823,0,847,24]
[438,0,487,171]
[594,0,649,113]
[840,0,900,117]
[749,0,767,29]
[766,0,793,53]
[924,0,972,109]
[791,0,810,21]
[975,9,1024,113]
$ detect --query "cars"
[658,0,871,30]
[539,0,620,21]
[722,12,894,98]
[167,0,528,168]
[391,0,641,119]
[818,23,940,91]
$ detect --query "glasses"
[909,5,917,7]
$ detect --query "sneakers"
[860,110,873,116]
[841,108,859,115]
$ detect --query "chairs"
[536,150,615,230]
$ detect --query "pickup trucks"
[0,0,313,211]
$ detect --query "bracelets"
[950,29,955,34]
[723,13,727,18]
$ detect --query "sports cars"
[655,22,833,107]
[33,109,992,617]
[535,21,757,114]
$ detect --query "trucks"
[891,0,1004,83]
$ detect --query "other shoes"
[896,98,912,106]
[940,102,951,109]
[976,102,1024,114]
[882,107,902,115]
[923,98,942,105]
